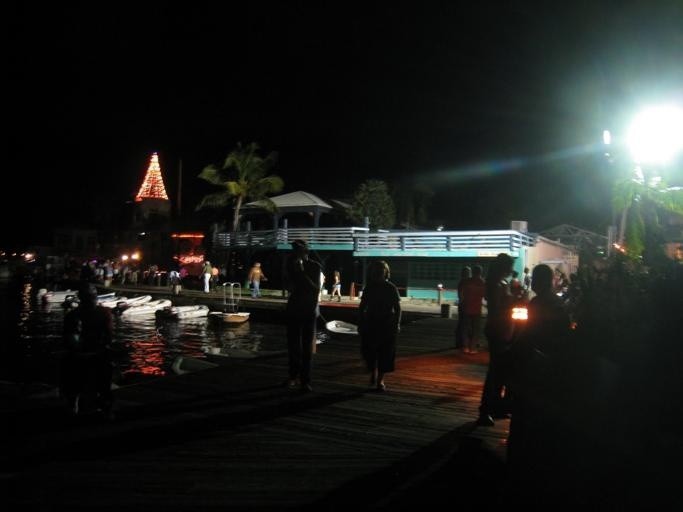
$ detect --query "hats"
[489,254,518,277]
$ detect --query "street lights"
[122,232,146,261]
[1,251,34,259]
[603,101,681,169]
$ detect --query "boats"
[326,319,359,335]
[112,298,172,321]
[210,310,250,323]
[38,289,152,308]
[155,304,210,322]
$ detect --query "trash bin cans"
[441,304,451,318]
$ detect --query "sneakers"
[495,412,512,419]
[287,378,312,393]
[369,374,385,390]
[456,345,480,354]
[479,414,494,426]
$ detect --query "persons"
[20,254,168,288]
[328,270,341,302]
[246,261,268,299]
[508,270,524,298]
[282,238,321,395]
[169,271,184,296]
[196,261,214,293]
[499,263,571,483]
[454,267,472,347]
[474,253,530,424]
[354,260,402,391]
[456,264,487,354]
[60,281,113,403]
